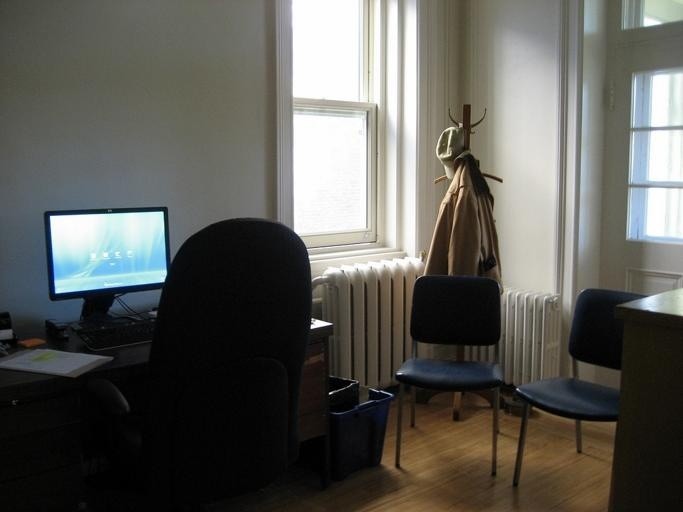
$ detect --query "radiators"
[320,256,561,404]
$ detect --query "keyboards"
[76,317,157,351]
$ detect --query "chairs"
[395,275,505,476]
[83,216,314,512]
[512,288,650,488]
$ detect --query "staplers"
[45,319,69,340]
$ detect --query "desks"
[0,311,335,511]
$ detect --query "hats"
[433,121,466,181]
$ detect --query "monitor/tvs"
[44,206,171,332]
